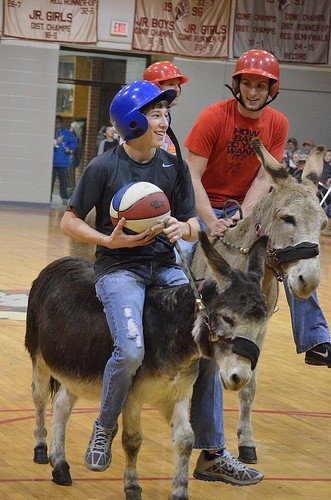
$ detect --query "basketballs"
[110,181,171,235]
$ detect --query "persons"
[143,61,189,156]
[60,80,265,487]
[50,115,83,205]
[281,137,331,216]
[96,126,119,157]
[173,49,331,369]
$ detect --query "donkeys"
[23,230,271,500]
[189,136,326,465]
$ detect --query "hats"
[287,138,298,147]
[296,155,307,164]
[303,138,315,146]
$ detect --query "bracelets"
[182,221,192,241]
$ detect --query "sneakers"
[305,342,331,364]
[193,450,264,488]
[84,421,118,472]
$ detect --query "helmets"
[143,63,190,88]
[109,80,178,139]
[224,50,279,97]
